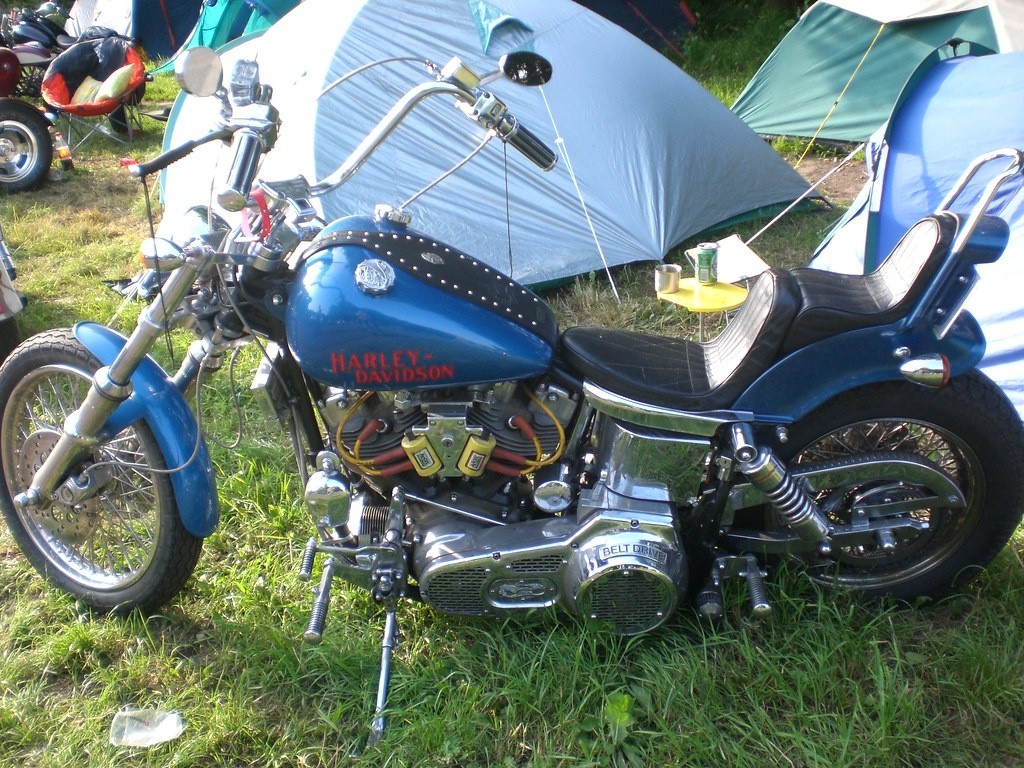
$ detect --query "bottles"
[55,132,74,171]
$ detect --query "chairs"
[41,36,146,154]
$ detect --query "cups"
[120,158,138,166]
[655,264,682,294]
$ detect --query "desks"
[658,275,751,341]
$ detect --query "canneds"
[696,242,718,285]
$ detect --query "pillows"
[70,61,136,103]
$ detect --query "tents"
[58,0,830,302]
[725,0,1024,418]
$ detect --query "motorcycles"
[0,41,1024,760]
[0,47,55,197]
